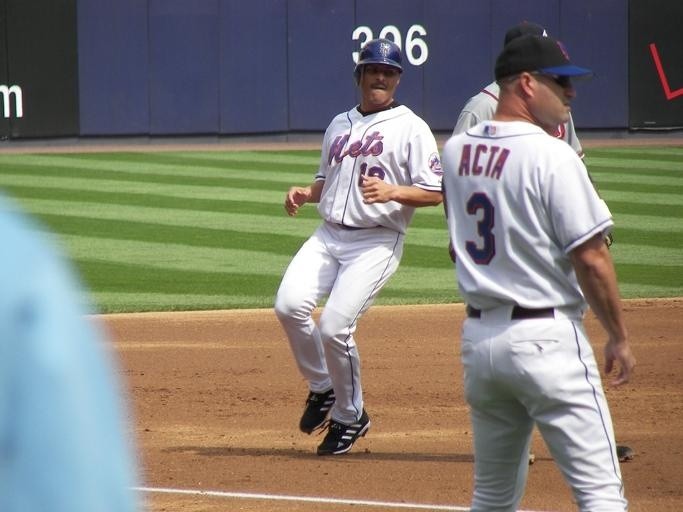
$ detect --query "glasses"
[508,71,569,89]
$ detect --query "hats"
[495,20,593,81]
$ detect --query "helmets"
[354,38,402,73]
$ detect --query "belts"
[466,303,553,319]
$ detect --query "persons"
[443,33,638,512]
[273,41,445,459]
[448,23,635,465]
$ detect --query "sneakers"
[300,388,336,435]
[317,407,371,455]
